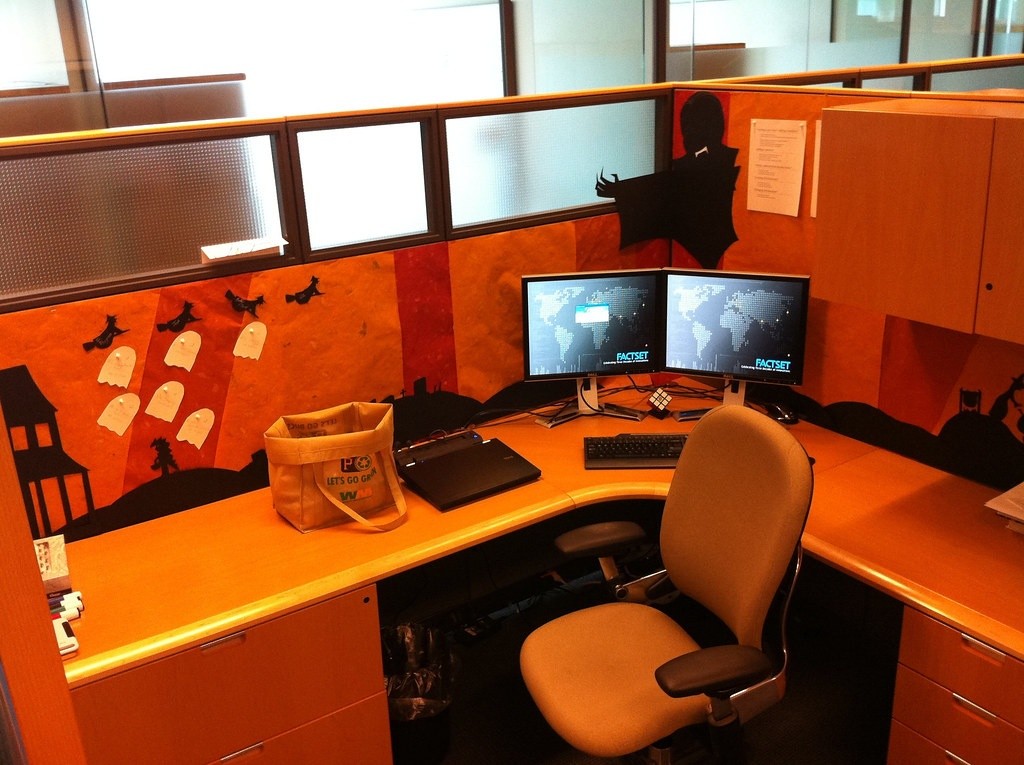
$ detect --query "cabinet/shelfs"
[809,97,1024,345]
[39,371,1024,765]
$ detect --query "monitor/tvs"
[522,268,664,429]
[663,266,811,422]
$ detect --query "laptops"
[402,437,541,512]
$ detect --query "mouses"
[767,401,799,424]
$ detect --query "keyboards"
[583,433,689,471]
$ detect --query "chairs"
[521,404,815,765]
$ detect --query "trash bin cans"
[379,622,456,765]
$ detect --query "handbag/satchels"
[264,402,408,531]
